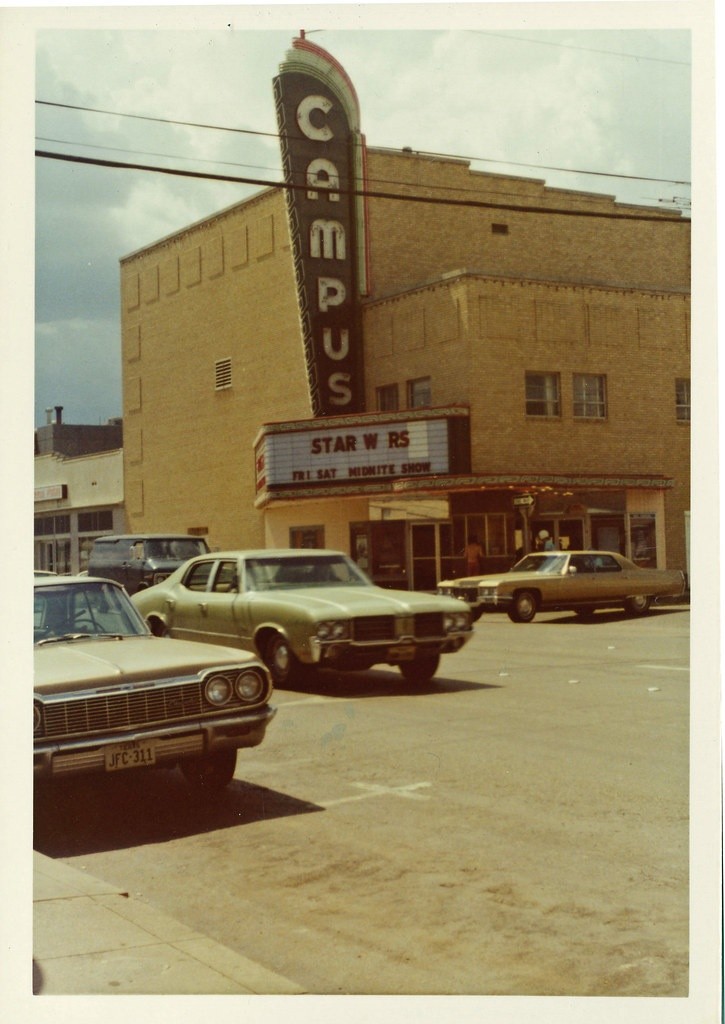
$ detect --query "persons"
[538,529,554,552]
[465,536,483,576]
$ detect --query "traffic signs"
[511,495,534,508]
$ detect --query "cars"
[435,549,687,625]
[33,576,278,795]
[87,532,236,599]
[128,548,478,687]
